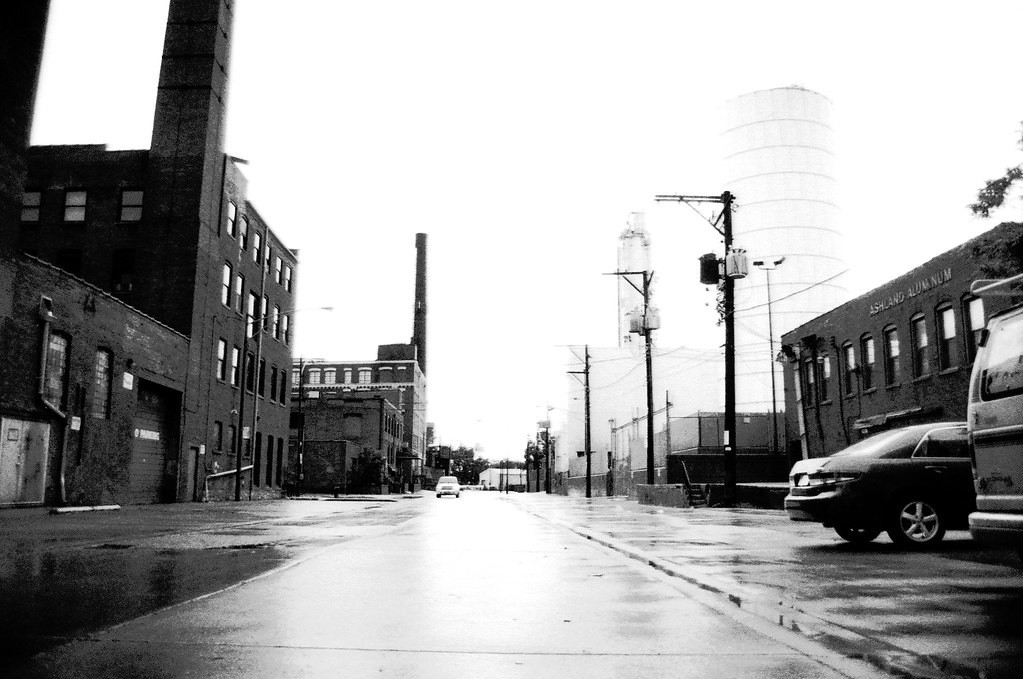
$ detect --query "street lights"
[753,257,786,454]
[296,358,314,496]
[235,306,332,502]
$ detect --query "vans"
[968,273,1023,562]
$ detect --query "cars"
[436,476,459,498]
[784,422,976,552]
